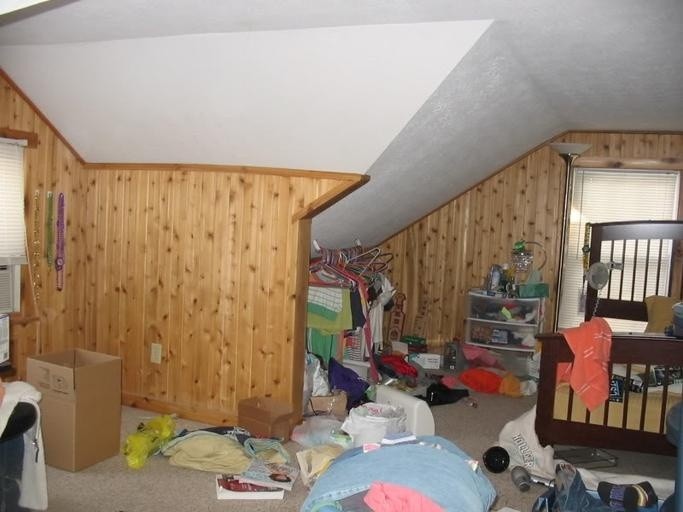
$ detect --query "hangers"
[309,245,394,287]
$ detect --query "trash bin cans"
[350,403,407,449]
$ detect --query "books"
[215,474,284,500]
[239,456,299,491]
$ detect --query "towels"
[554,316,612,412]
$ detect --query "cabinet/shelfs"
[465,287,545,360]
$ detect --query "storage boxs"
[238,396,294,446]
[307,388,348,417]
[26,346,123,472]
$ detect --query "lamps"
[586,261,609,290]
